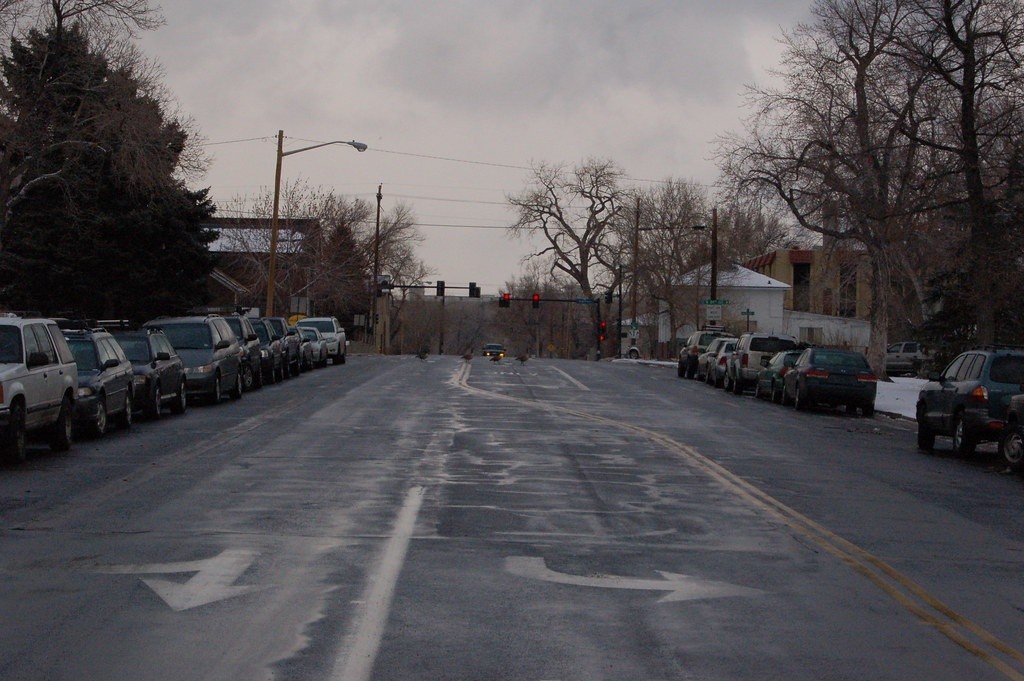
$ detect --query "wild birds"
[461,348,474,363]
[489,349,507,365]
[515,348,528,366]
[415,349,431,361]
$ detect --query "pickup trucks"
[886,340,941,377]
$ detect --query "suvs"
[677,330,734,379]
[61,329,136,442]
[140,313,245,407]
[296,316,346,366]
[915,344,1024,461]
[222,314,302,392]
[0,312,79,467]
[725,331,799,395]
[95,318,187,422]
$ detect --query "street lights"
[265,129,368,318]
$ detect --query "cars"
[754,351,803,404]
[289,325,315,373]
[482,343,507,357]
[782,345,878,416]
[996,395,1024,478]
[695,337,740,388]
[298,327,328,369]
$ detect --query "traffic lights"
[504,294,510,307]
[532,294,540,309]
[600,321,607,341]
[603,290,613,304]
